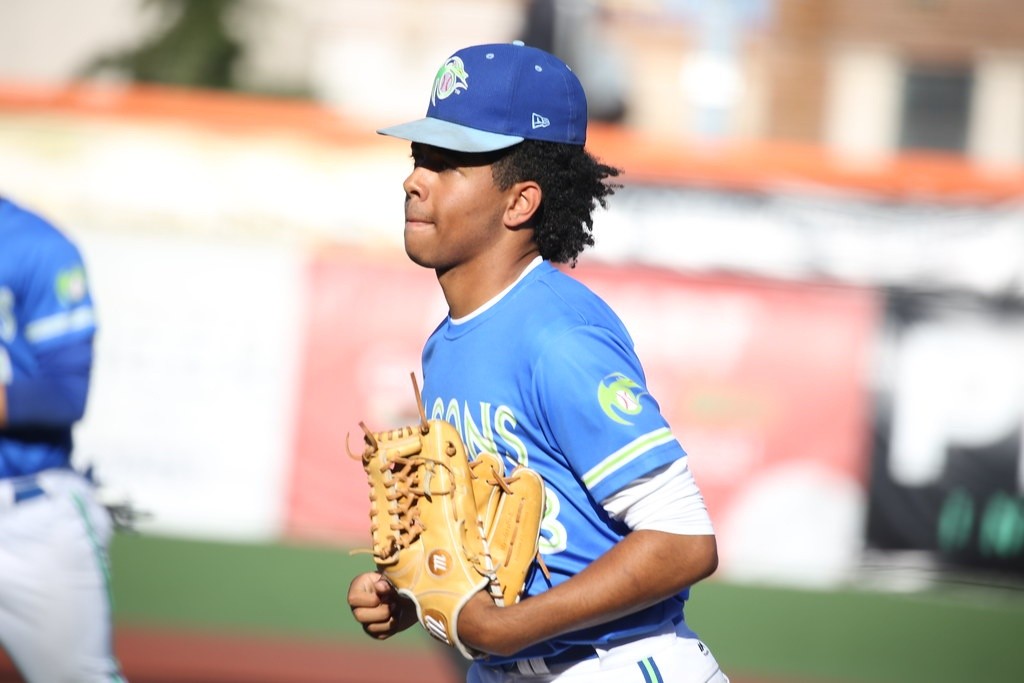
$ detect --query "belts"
[480,611,684,674]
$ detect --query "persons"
[347,39,728,681]
[0,195,126,681]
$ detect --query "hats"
[376,39,587,152]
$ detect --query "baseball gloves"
[363,419,546,662]
